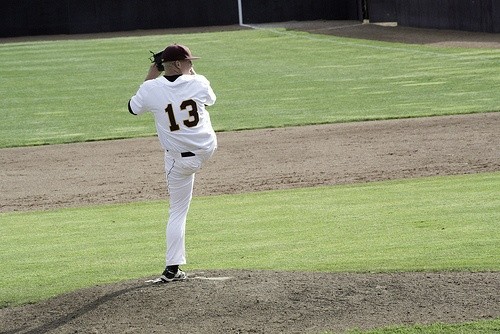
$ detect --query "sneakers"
[161,268,188,281]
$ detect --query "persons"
[128,43,218,282]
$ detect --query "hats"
[161,45,200,63]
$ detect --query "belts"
[181,151,195,157]
